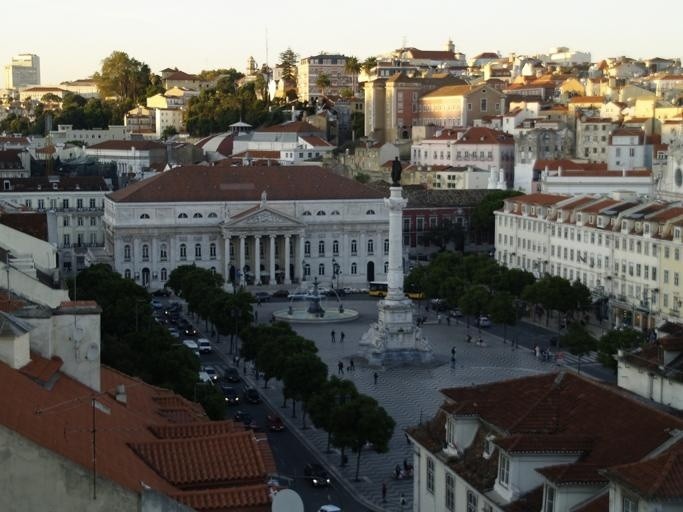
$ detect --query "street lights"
[333,267,342,296]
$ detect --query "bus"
[369,280,426,299]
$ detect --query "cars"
[255,286,369,300]
[431,297,489,326]
[152,294,283,439]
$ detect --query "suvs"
[304,461,330,488]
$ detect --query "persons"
[339,331,345,343]
[260,189,267,209]
[374,371,379,384]
[331,329,336,343]
[449,346,457,369]
[438,314,441,325]
[390,155,403,186]
[395,458,414,482]
[349,358,355,371]
[533,342,553,362]
[337,361,344,375]
[256,295,263,308]
[381,482,388,504]
[447,315,450,326]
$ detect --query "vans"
[317,505,341,511]
